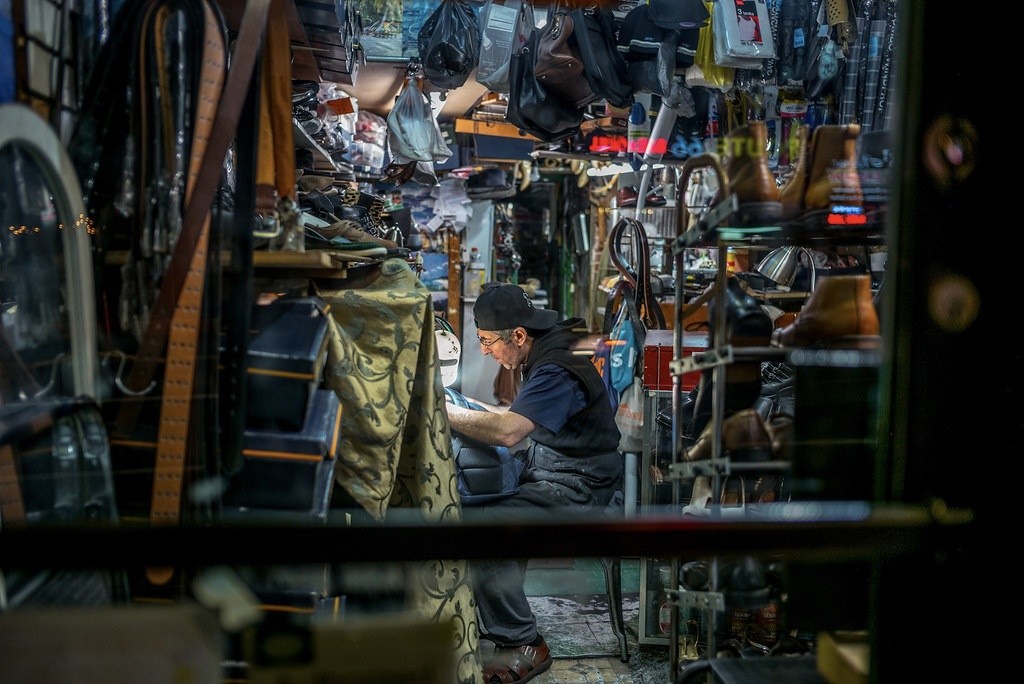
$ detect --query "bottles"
[659,577,672,633]
[469,248,480,264]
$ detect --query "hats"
[473,284,559,330]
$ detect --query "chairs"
[595,452,637,663]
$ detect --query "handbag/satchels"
[389,0,777,162]
[590,299,661,453]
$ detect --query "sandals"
[485,634,553,684]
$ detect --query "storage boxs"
[239,390,343,511]
[243,311,327,432]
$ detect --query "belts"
[602,214,667,343]
[63,1,313,587]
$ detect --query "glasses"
[476,331,515,349]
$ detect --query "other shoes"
[282,151,404,282]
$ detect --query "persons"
[738,5,761,41]
[447,284,620,684]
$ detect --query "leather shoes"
[669,122,884,466]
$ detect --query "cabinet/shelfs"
[482,0,905,684]
[0,0,462,684]
